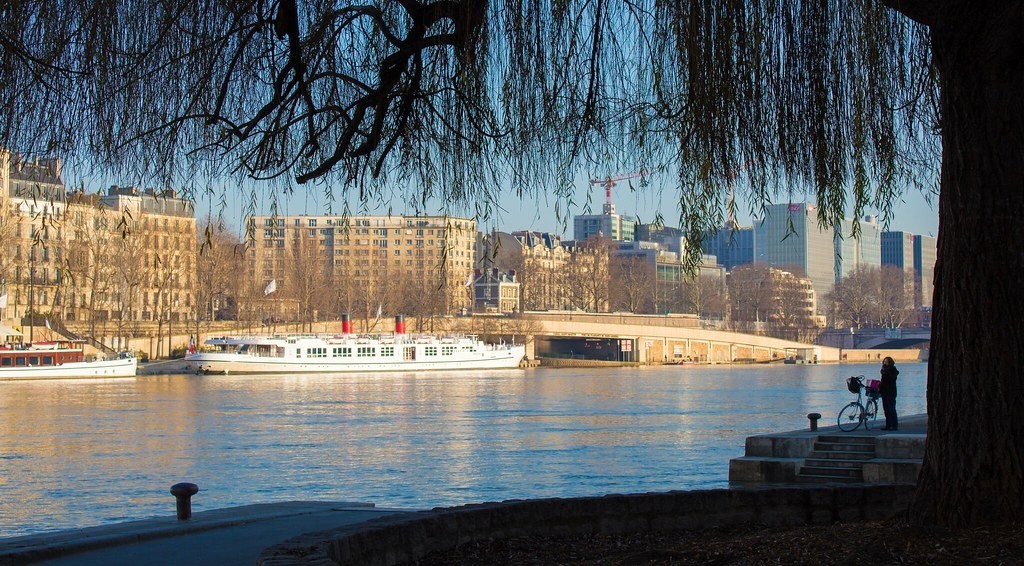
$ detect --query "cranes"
[591,167,660,214]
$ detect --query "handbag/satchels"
[866,379,880,390]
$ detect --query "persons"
[878,356,899,431]
[571,350,573,358]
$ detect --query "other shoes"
[888,427,897,431]
[880,427,889,431]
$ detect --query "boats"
[183,329,527,375]
[0,293,138,381]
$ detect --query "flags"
[45,319,51,329]
[264,279,276,295]
[466,272,473,285]
[189,335,194,354]
[0,295,7,308]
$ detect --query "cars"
[673,353,691,363]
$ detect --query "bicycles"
[835,374,881,432]
